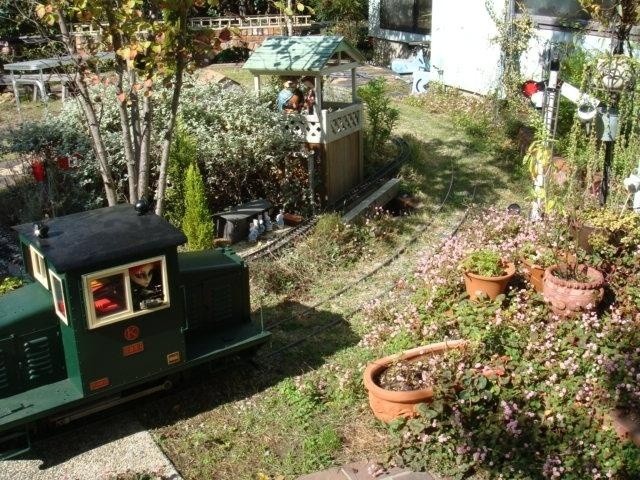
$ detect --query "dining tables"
[572,92,640,177]
[572,205,621,255]
[578,0,640,91]
[519,241,576,293]
[364,340,486,427]
[522,127,607,320]
[459,249,515,303]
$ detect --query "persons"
[130,263,161,311]
[276,75,323,115]
